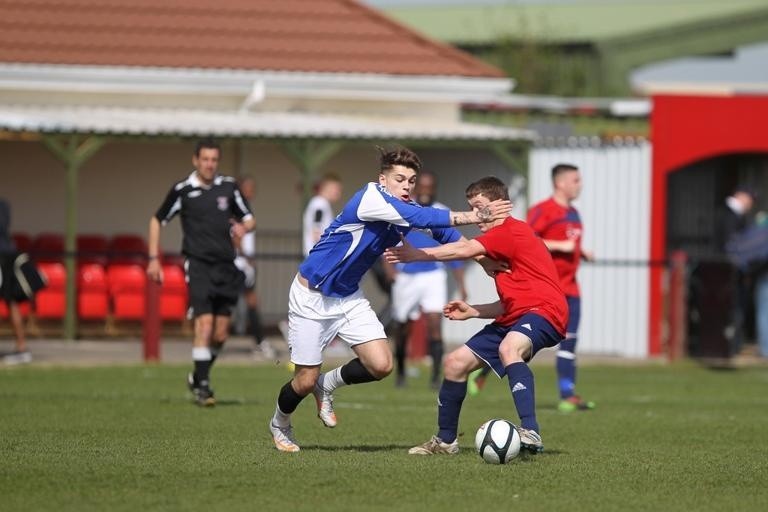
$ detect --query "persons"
[382,169,467,391]
[382,176,570,458]
[268,146,513,454]
[467,164,597,412]
[145,140,256,409]
[704,181,762,369]
[234,173,280,360]
[302,171,343,261]
[0,197,34,366]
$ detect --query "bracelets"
[148,254,160,259]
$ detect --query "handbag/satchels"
[5,251,45,301]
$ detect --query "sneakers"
[396,374,403,386]
[311,374,338,428]
[409,435,459,454]
[5,349,30,365]
[188,372,216,407]
[517,425,543,454]
[270,417,301,452]
[558,395,595,412]
[430,375,439,387]
[468,366,491,397]
[256,339,278,360]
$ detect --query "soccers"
[475,418,521,464]
